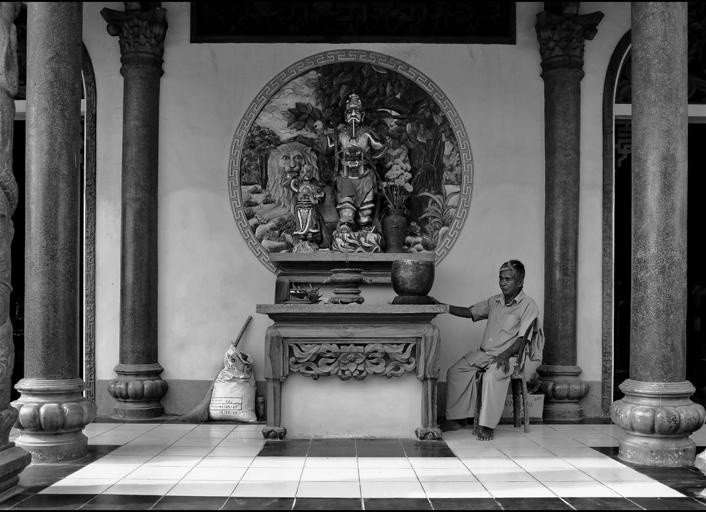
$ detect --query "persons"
[427,259,541,441]
[289,165,326,243]
[313,91,385,231]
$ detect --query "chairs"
[471,317,538,436]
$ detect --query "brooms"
[178,315,252,424]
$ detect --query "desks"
[255,303,449,441]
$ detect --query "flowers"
[378,158,416,211]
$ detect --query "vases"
[381,206,407,253]
